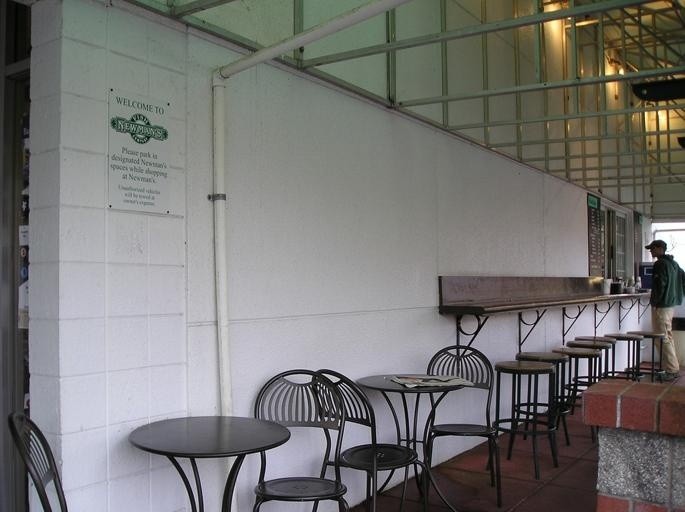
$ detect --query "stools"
[485,330,667,481]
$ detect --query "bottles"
[618,275,642,293]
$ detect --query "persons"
[645,239,685,382]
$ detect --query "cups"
[601,278,612,295]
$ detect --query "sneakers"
[655,371,678,380]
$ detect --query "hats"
[645,240,666,250]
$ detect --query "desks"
[357,372,466,511]
[438,272,654,360]
[130,414,292,511]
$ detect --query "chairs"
[252,368,351,511]
[6,409,69,511]
[424,342,503,508]
[311,368,418,512]
